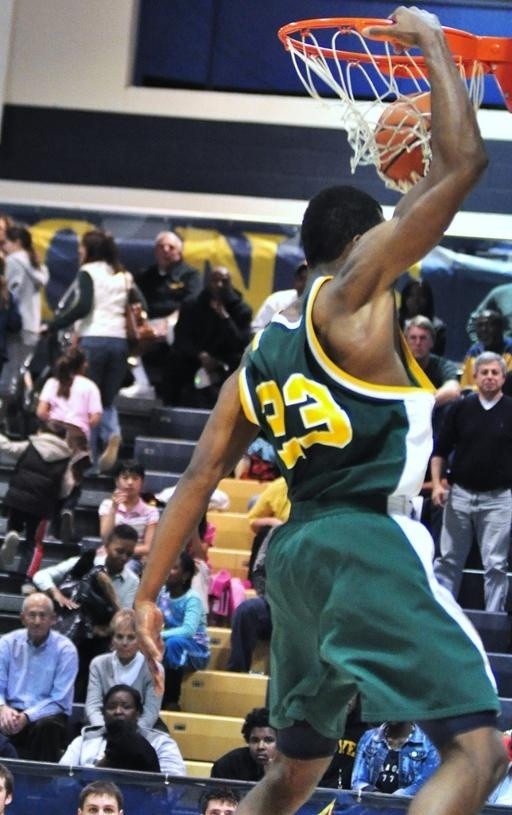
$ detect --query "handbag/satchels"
[118,271,161,354]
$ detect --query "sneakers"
[0,528,22,567]
[96,433,121,474]
[59,509,75,542]
[116,384,158,402]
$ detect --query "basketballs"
[373,92,432,192]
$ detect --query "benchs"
[144,396,273,815]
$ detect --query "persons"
[318,692,442,797]
[461,310,510,396]
[32,524,140,703]
[0,227,50,440]
[35,347,102,541]
[133,6,511,815]
[37,230,148,476]
[0,765,14,812]
[187,510,216,565]
[399,277,461,407]
[59,685,187,776]
[1,420,75,595]
[228,476,292,674]
[156,551,210,712]
[0,593,79,762]
[252,261,307,338]
[430,351,512,615]
[85,607,165,730]
[98,458,160,561]
[202,782,240,815]
[118,231,253,408]
[211,708,276,782]
[77,780,124,814]
